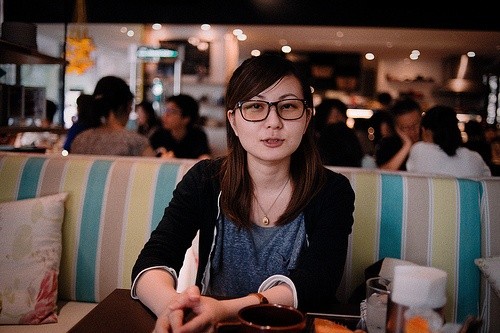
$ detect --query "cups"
[237,303,306,333]
[365,276,393,333]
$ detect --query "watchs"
[248,293,269,304]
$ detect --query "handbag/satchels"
[359,256,413,333]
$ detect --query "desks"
[66,288,361,333]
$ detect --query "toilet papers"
[392,265,448,309]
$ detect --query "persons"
[128,53,356,333]
[47,75,500,178]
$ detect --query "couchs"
[0,152,500,333]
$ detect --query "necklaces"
[251,176,291,225]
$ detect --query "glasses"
[231,98,308,122]
[400,122,421,132]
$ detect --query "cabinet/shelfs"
[146,35,239,158]
[0,39,71,134]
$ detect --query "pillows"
[0,192,69,325]
